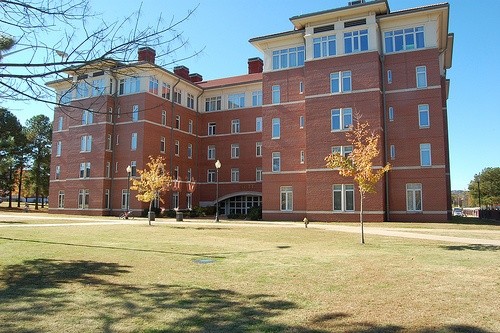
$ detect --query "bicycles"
[119,211,134,220]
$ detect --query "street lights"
[126,165,132,213]
[476,176,481,207]
[214,159,222,222]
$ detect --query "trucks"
[452,207,463,217]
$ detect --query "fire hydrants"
[303,217,309,228]
